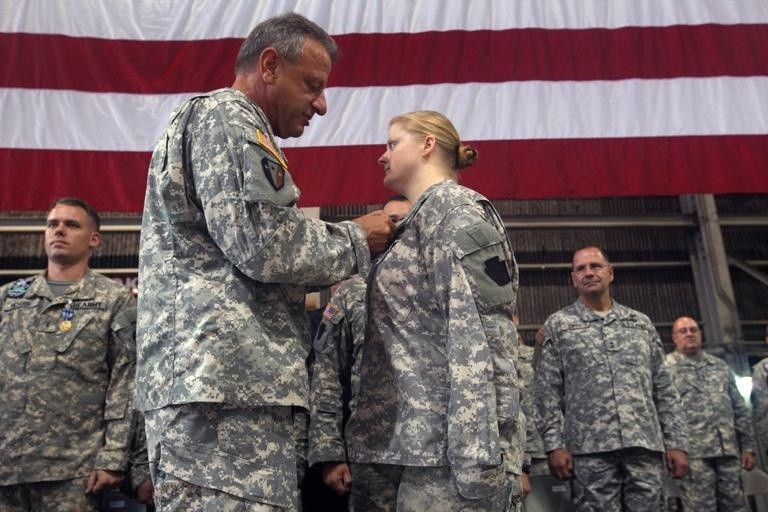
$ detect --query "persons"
[136,9,398,511]
[510,309,571,512]
[663,314,759,512]
[344,109,521,511]
[298,275,352,511]
[750,328,768,479]
[122,409,158,511]
[532,245,689,512]
[0,197,137,512]
[307,194,413,511]
